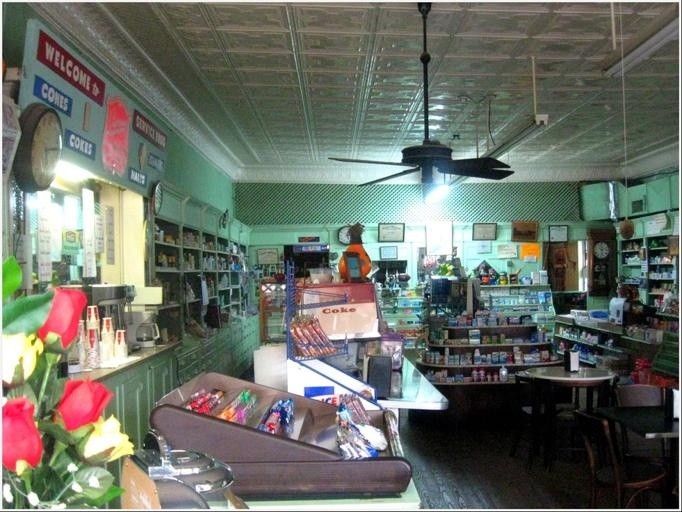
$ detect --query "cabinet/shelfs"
[584,225,618,300]
[481,283,559,348]
[644,232,680,380]
[550,311,622,373]
[379,284,426,350]
[408,322,572,434]
[95,180,263,457]
[616,234,652,349]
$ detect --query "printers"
[608,297,657,327]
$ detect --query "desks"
[518,363,623,484]
[604,405,679,486]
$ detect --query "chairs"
[602,382,678,509]
[513,374,575,468]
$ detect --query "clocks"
[336,224,354,246]
[594,241,612,262]
[11,102,65,195]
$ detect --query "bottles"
[471,366,508,382]
[338,223,371,284]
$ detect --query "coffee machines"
[80,282,135,354]
[126,286,164,350]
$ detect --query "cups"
[74,306,129,371]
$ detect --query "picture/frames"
[510,219,539,242]
[471,222,499,242]
[378,222,406,243]
[548,224,569,243]
[378,245,399,261]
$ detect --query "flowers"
[3,247,139,508]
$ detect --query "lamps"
[597,4,678,85]
[438,52,550,191]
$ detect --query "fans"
[324,2,516,202]
